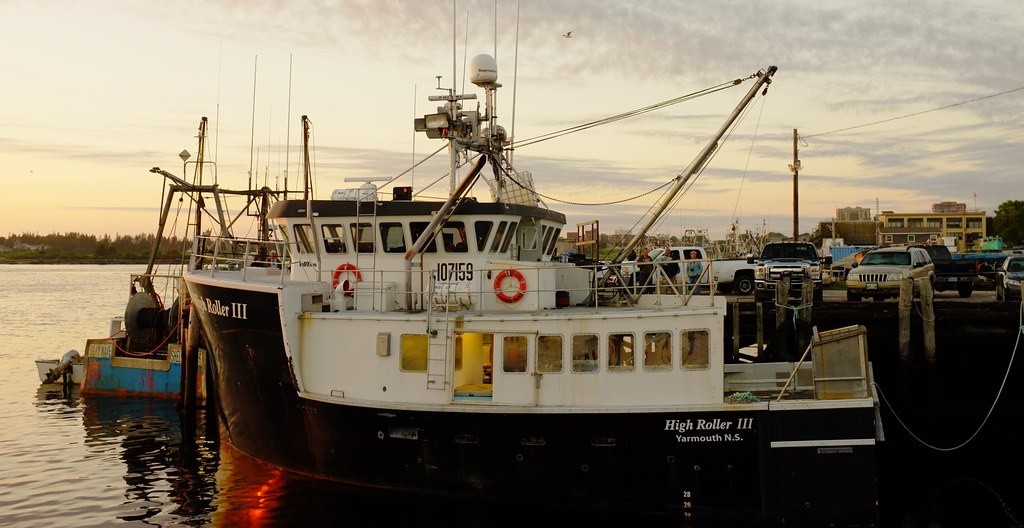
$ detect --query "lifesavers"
[333,264,362,296]
[494,270,527,304]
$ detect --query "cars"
[995,255,1024,302]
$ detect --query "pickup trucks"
[619,246,756,295]
[917,244,979,298]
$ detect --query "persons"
[687,251,703,294]
[660,247,679,294]
[267,250,282,270]
[251,256,264,267]
[638,248,654,294]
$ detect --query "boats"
[182,0,884,528]
[35,350,86,388]
[77,115,311,403]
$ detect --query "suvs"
[746,239,833,302]
[845,245,935,303]
